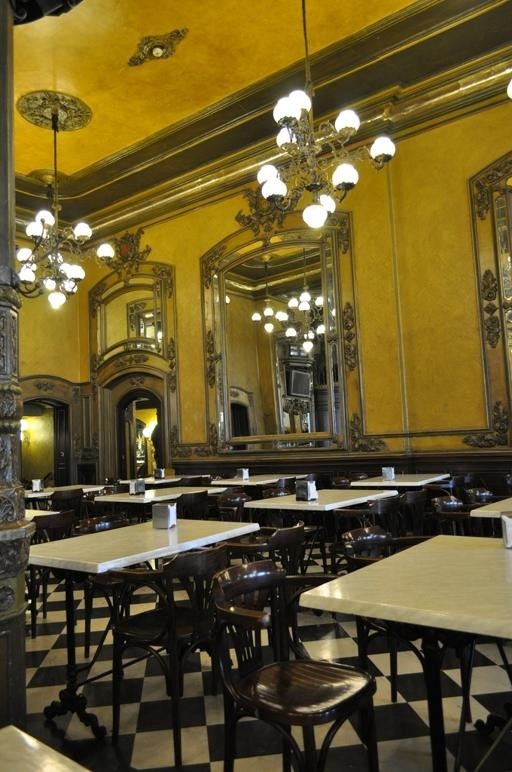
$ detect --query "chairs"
[210,561,380,769]
[103,544,228,768]
[16,461,511,638]
[340,524,437,702]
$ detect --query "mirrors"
[197,208,366,459]
[86,258,183,366]
[464,147,512,448]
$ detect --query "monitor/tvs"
[290,370,312,398]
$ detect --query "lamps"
[251,0,401,235]
[248,247,342,357]
[12,88,116,312]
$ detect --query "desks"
[297,534,511,770]
